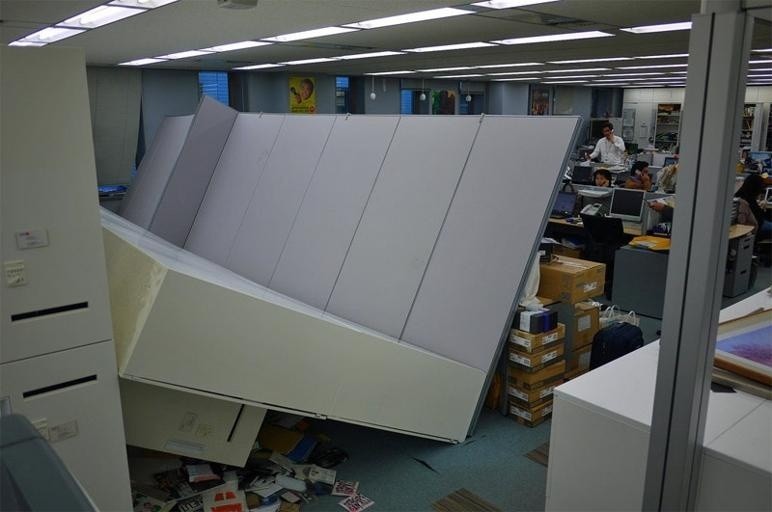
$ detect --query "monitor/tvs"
[572,166,592,185]
[609,188,646,222]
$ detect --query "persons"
[585,122,765,238]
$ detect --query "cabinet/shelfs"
[739,101,765,158]
[0,46,137,512]
[653,110,683,153]
[722,234,756,298]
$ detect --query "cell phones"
[635,169,642,176]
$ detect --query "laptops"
[550,191,578,219]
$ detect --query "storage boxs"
[501,252,609,429]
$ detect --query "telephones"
[580,203,602,217]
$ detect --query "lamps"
[369,76,472,102]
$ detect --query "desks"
[546,214,642,260]
[628,221,757,252]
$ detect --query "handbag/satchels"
[598,304,639,329]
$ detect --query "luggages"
[589,322,645,371]
